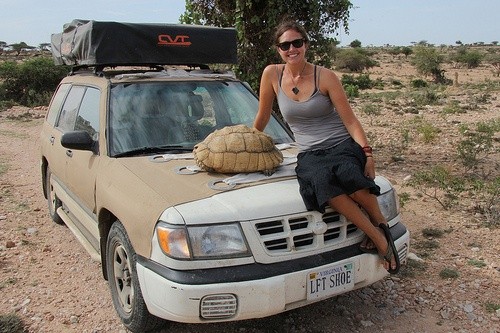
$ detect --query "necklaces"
[280,64,308,95]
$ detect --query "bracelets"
[362,145,373,157]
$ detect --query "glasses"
[276,39,306,51]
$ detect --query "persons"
[251,21,400,275]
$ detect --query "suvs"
[36,19,409,332]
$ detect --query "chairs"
[168,96,211,144]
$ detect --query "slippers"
[378,223,401,274]
